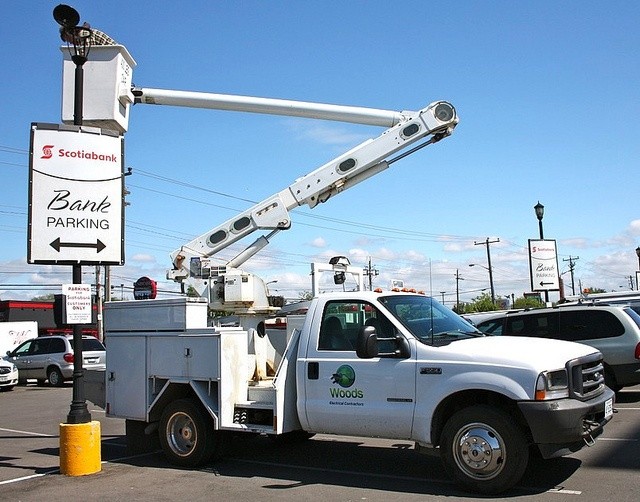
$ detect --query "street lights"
[52,5,93,118]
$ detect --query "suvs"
[2,333,109,385]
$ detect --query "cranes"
[133,84,459,378]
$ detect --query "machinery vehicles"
[60,44,619,497]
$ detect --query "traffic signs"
[25,120,131,475]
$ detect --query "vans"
[470,305,639,401]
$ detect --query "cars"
[0,362,18,388]
